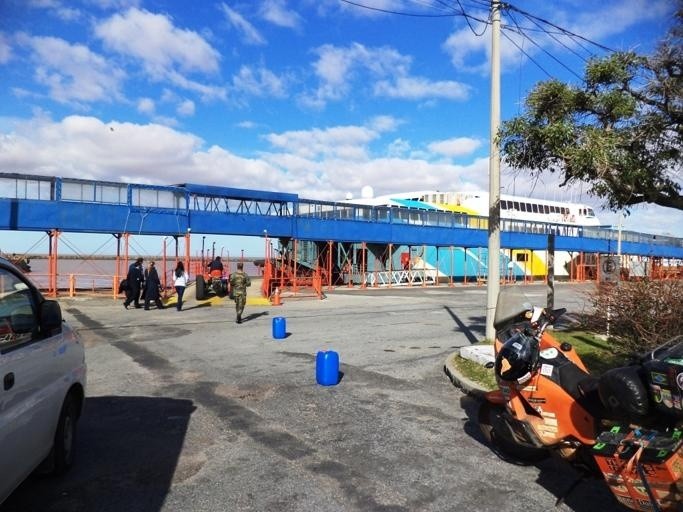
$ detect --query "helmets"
[496,332,538,381]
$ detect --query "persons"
[119,257,164,311]
[228,262,251,323]
[171,261,189,312]
[205,256,224,292]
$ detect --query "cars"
[0,257,88,510]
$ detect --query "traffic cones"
[270,286,283,305]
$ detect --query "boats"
[252,184,599,285]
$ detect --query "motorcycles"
[474,304,682,512]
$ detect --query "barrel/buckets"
[272,317,285,338]
[316,351,339,386]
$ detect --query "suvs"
[195,270,236,300]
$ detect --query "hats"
[237,263,243,267]
